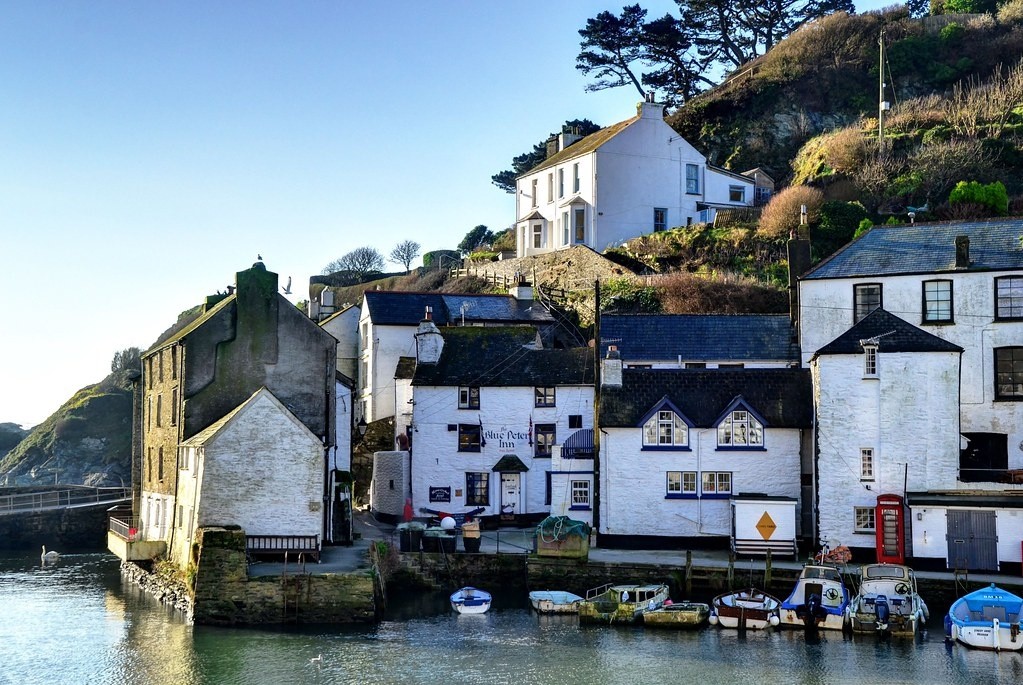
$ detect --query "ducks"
[310,654,324,662]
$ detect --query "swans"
[40,545,61,558]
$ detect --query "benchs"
[243,532,322,562]
[729,537,801,559]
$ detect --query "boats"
[844,562,930,638]
[578,582,710,624]
[711,587,782,629]
[528,590,585,613]
[449,586,492,614]
[778,541,850,630]
[944,583,1023,653]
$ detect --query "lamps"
[342,391,368,437]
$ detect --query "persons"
[396,431,409,450]
[403,498,415,521]
